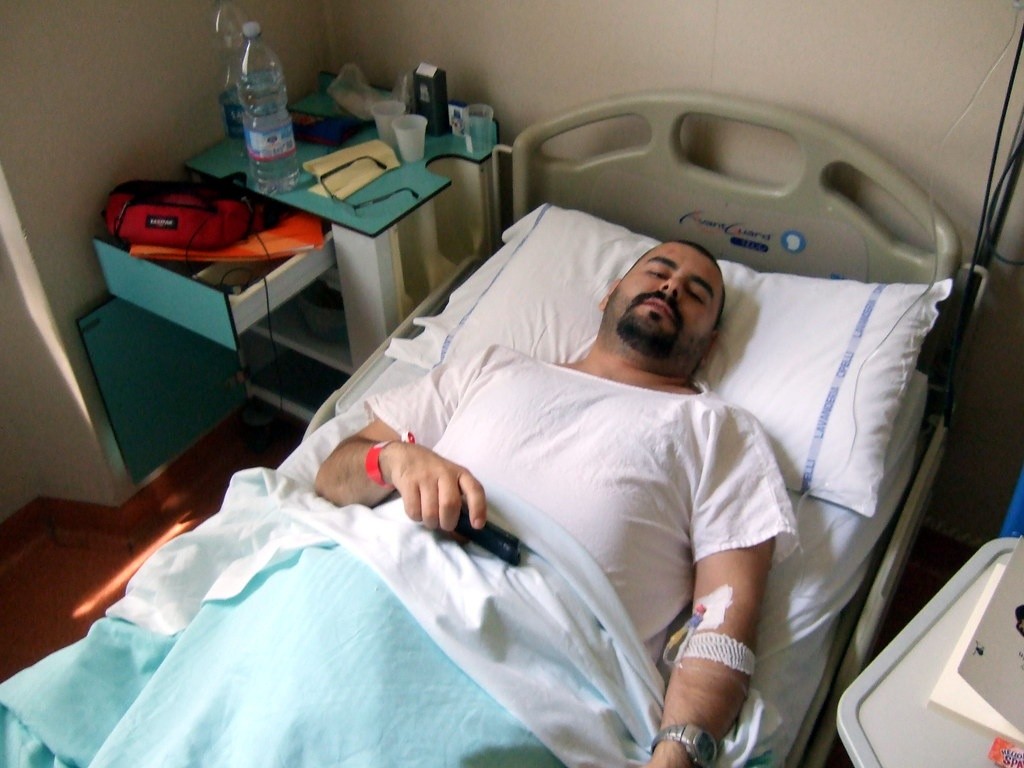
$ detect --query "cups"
[461,103,494,153]
[391,114,428,162]
[372,99,406,148]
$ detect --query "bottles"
[236,20,300,195]
[210,0,250,167]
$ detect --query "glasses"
[320,155,419,215]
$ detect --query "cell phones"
[456,506,523,566]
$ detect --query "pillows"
[386,202,953,520]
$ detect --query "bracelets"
[651,723,718,768]
[365,431,414,490]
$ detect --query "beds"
[1,90,961,766]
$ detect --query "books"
[927,535,1024,749]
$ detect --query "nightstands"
[73,70,506,479]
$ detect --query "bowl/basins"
[295,280,348,344]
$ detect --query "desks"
[836,536,1023,765]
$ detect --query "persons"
[316,240,800,768]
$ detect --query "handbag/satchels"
[106,171,254,250]
